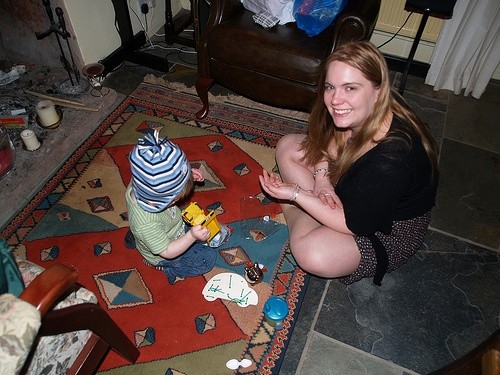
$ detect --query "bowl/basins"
[35,107,63,129]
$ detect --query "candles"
[36,99,59,126]
[20,129,40,151]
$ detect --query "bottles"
[263,295,289,330]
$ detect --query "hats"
[127,130,192,214]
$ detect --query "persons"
[259,42,438,287]
[125,129,217,285]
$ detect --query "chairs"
[190,0,381,120]
[0,258,141,375]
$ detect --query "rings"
[325,194,330,196]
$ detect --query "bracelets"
[290,183,301,201]
[313,168,330,178]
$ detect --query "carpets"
[0,73,313,375]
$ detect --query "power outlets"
[137,0,154,15]
